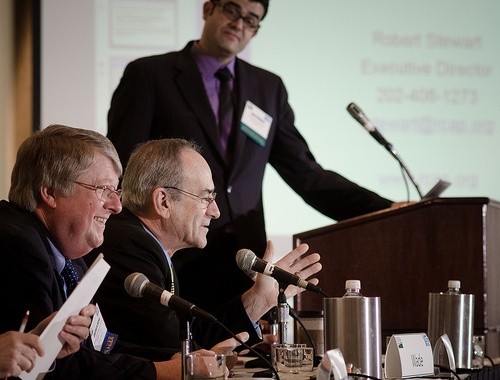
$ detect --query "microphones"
[235,249,321,292]
[347,103,392,149]
[124,273,196,311]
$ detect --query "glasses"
[74,181,123,201]
[163,186,217,204]
[221,4,258,32]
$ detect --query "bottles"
[446,280,461,294]
[342,279,363,297]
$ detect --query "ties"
[62,260,81,299]
[213,67,239,163]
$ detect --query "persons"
[0,124,249,380]
[84,139,322,351]
[0,304,96,378]
[106,0,407,259]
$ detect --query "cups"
[271,343,314,377]
[321,296,383,380]
[428,293,474,374]
[186,353,224,380]
[474,336,486,368]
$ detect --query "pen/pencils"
[3,309,30,380]
[235,360,244,365]
